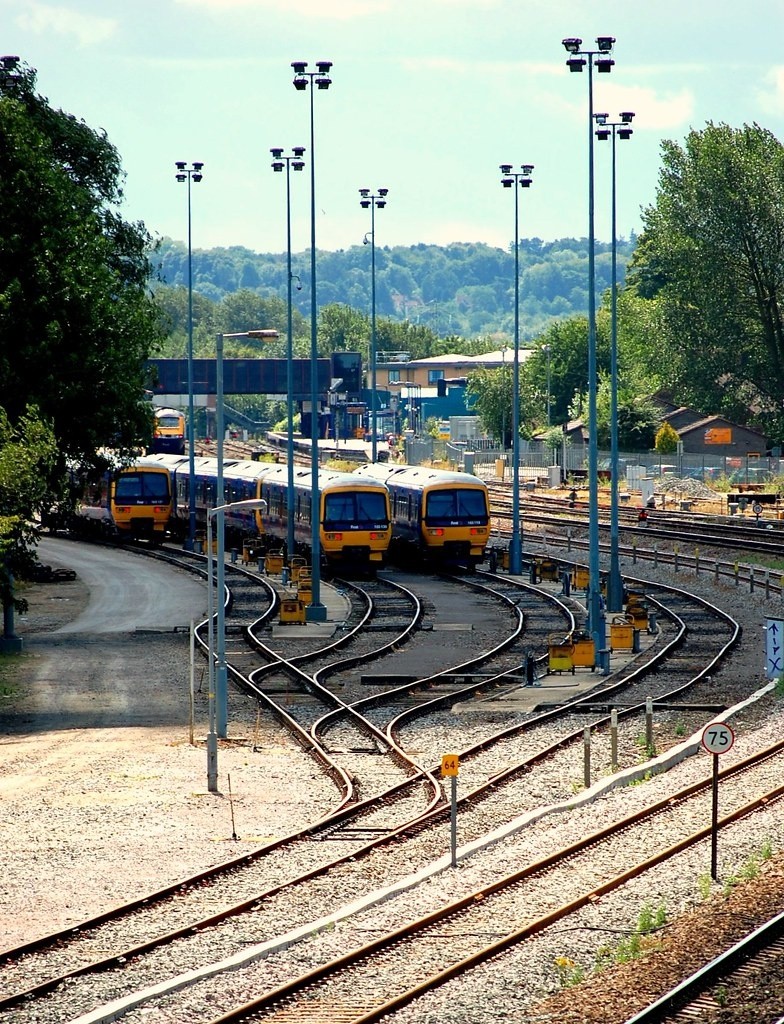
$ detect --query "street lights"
[206,498,269,792]
[499,165,536,574]
[176,160,206,549]
[562,36,617,674]
[593,112,636,614]
[216,329,280,739]
[359,190,389,457]
[287,58,333,617]
[272,145,306,562]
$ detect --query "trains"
[146,451,395,582]
[149,405,186,455]
[353,460,492,569]
[61,457,174,545]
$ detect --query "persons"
[638,510,647,522]
